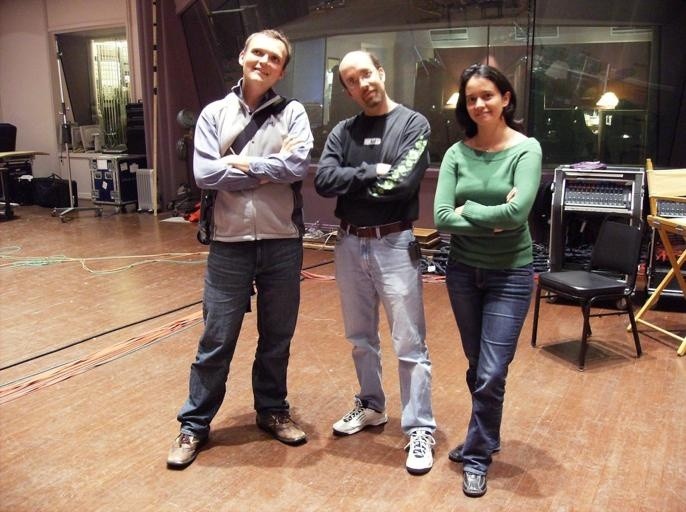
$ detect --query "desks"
[626,215,686,357]
[0,150,51,221]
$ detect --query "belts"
[340,220,413,238]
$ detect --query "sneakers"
[463,464,488,497]
[166,428,209,467]
[256,410,306,444]
[332,396,388,435]
[405,427,434,473]
[448,439,501,462]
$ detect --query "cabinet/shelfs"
[87,154,146,214]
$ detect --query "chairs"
[531,212,646,372]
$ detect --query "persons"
[309,48,440,478]
[161,25,317,469]
[426,60,545,500]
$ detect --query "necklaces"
[476,139,497,154]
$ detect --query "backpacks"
[197,189,217,246]
